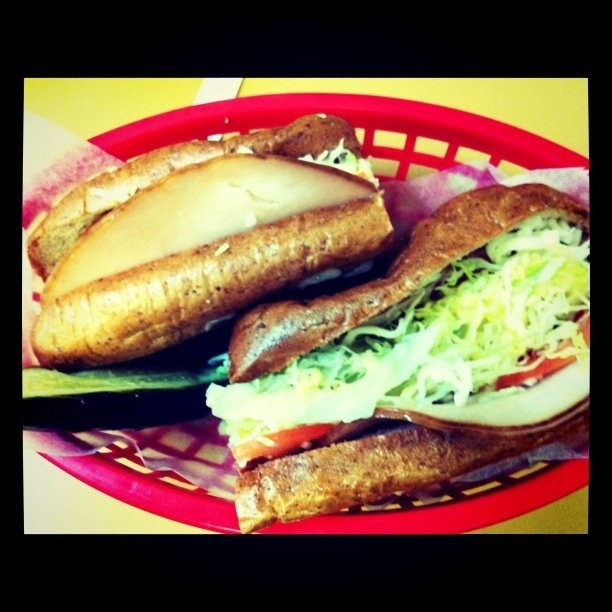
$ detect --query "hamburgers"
[205,184,591,537]
[28,113,396,368]
